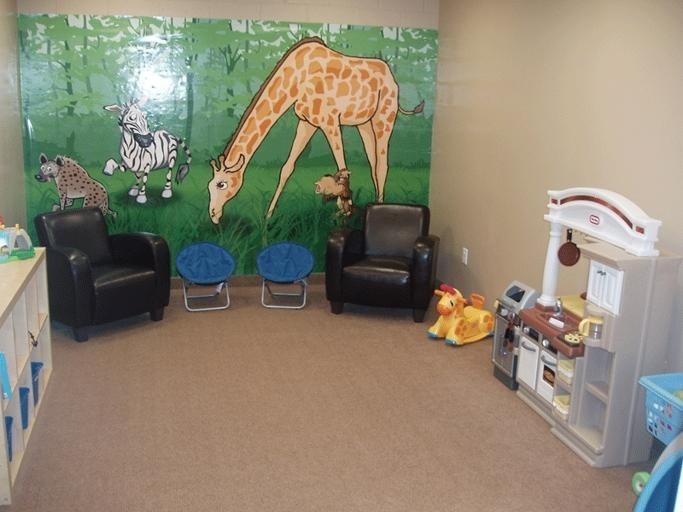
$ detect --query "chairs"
[34,205,170,342]
[325,203,440,323]
[255,242,315,309]
[175,242,235,311]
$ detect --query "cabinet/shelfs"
[0,247,53,505]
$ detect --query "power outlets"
[462,247,468,266]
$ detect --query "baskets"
[638,373,683,446]
